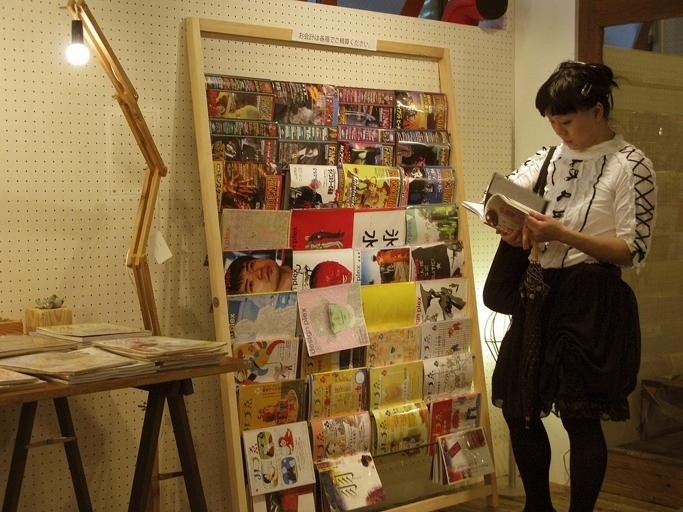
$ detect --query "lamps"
[59,1,169,336]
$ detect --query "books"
[461,172,550,245]
[0,322,231,391]
[205,75,498,511]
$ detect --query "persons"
[480,59,658,511]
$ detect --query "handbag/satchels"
[483,237,531,315]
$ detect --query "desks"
[0,323,255,512]
